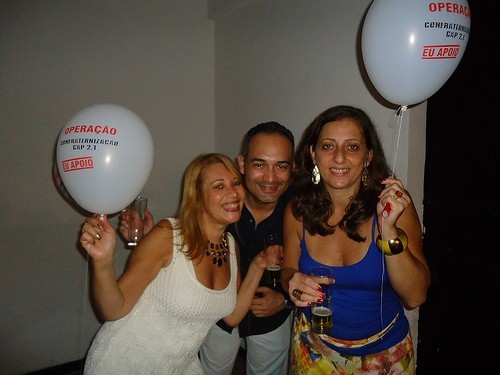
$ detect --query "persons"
[119,121,297,375]
[281,106,430,375]
[79,153,284,375]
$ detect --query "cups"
[310,267,334,334]
[265,234,282,288]
[127,197,148,247]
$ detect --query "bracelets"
[376,228,408,255]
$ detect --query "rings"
[292,289,302,300]
[393,190,403,199]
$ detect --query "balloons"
[360,0,471,111]
[57,104,153,215]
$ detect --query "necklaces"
[206,232,227,267]
